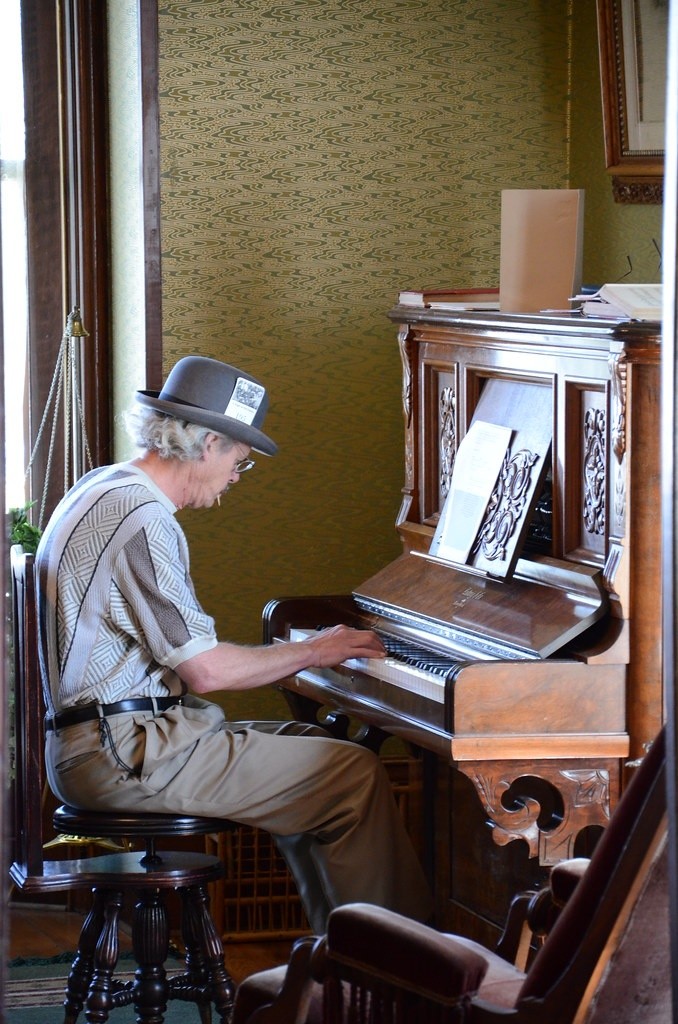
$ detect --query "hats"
[136,356,280,457]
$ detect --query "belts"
[45,695,184,731]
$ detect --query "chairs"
[1,544,240,1023]
[219,721,676,1023]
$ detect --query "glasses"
[231,440,255,473]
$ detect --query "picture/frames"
[595,0,664,204]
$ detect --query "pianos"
[262,288,661,970]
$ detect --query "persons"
[30,355,438,954]
